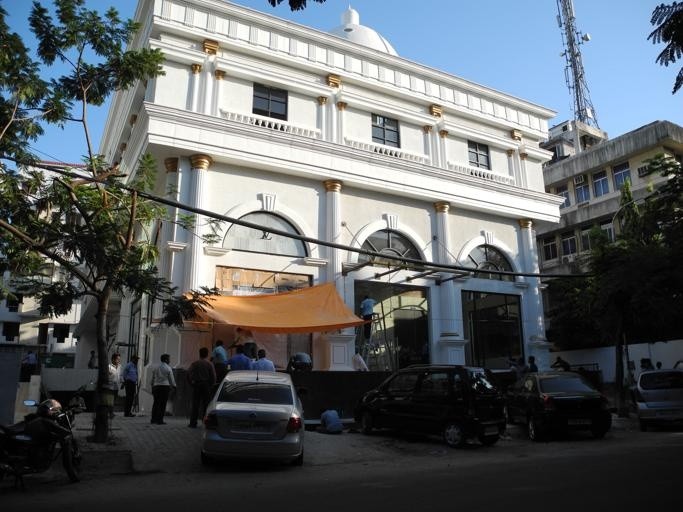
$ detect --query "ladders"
[362,312,392,372]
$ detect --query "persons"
[550,356,571,371]
[503,355,538,381]
[315,407,346,434]
[21,350,38,381]
[68,391,87,413]
[108,326,313,428]
[638,362,663,379]
[360,295,377,339]
[402,341,427,362]
[88,350,99,368]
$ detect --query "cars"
[350,362,507,447]
[502,370,612,441]
[200,369,304,467]
[627,367,683,432]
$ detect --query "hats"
[131,355,140,360]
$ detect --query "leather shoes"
[126,413,136,417]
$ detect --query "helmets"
[38,400,63,417]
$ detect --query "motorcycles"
[0,398,81,488]
